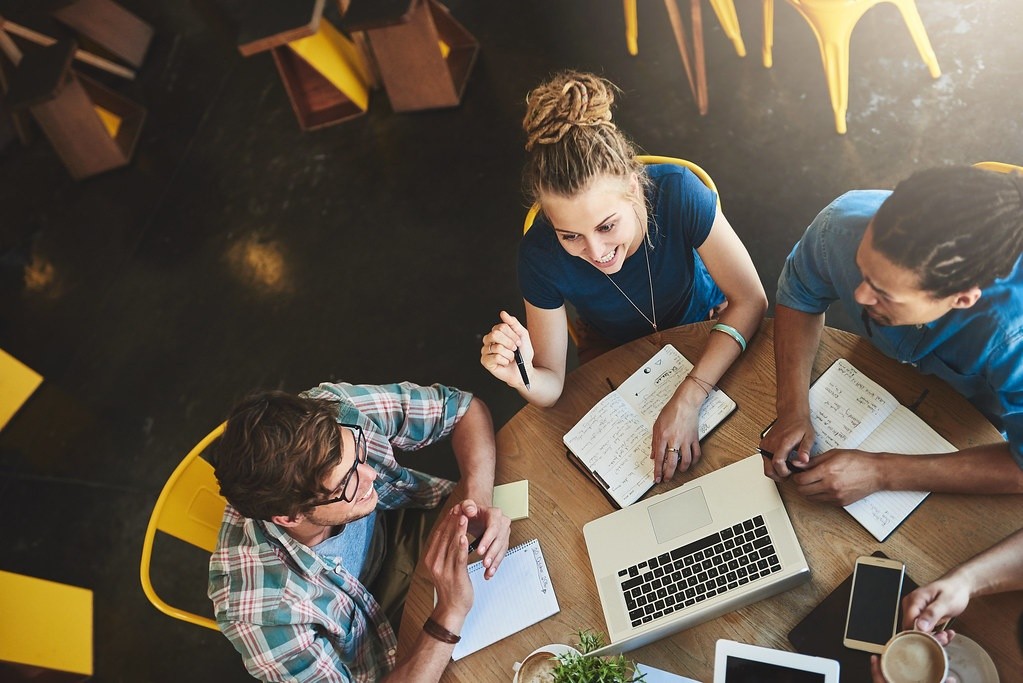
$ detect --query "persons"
[207,379,512,683]
[869,524,1022,682]
[757,163,1023,512]
[480,71,769,487]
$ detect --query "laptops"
[582,452,812,657]
[713,638,840,683]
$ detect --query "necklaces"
[604,205,658,332]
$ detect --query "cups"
[879,619,948,683]
[512,652,564,683]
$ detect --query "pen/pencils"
[513,346,530,392]
[468,533,483,555]
[756,447,805,473]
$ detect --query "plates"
[928,631,999,683]
[512,643,583,682]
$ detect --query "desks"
[395,315,1023,683]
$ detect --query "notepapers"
[493,479,529,522]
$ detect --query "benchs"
[0,37,150,182]
[236,1,483,134]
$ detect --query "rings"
[667,448,680,454]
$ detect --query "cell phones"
[843,556,907,654]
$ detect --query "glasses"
[303,423,367,508]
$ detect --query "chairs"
[140,416,230,634]
[519,0,1023,364]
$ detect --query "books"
[433,537,561,662]
[760,357,961,543]
[562,343,738,510]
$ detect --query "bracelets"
[709,322,747,353]
[685,371,715,399]
[423,616,461,645]
